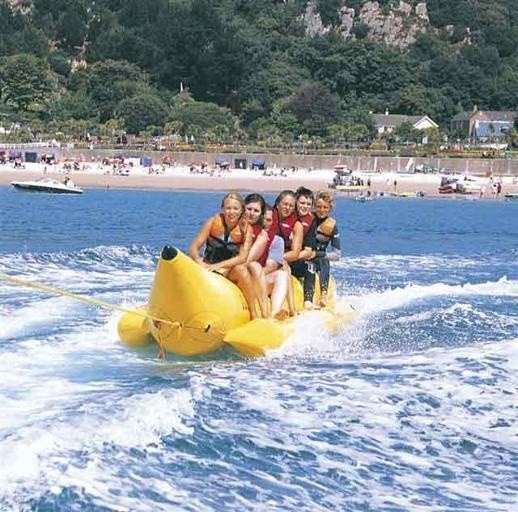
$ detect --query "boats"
[10,177,83,193]
[118,244,359,356]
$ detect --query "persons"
[491,182,501,199]
[190,160,230,177]
[189,187,341,321]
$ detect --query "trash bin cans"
[25,153,36,162]
[215,159,224,165]
[235,159,246,168]
[252,161,264,169]
[144,157,153,167]
[505,151,513,160]
[432,167,438,174]
